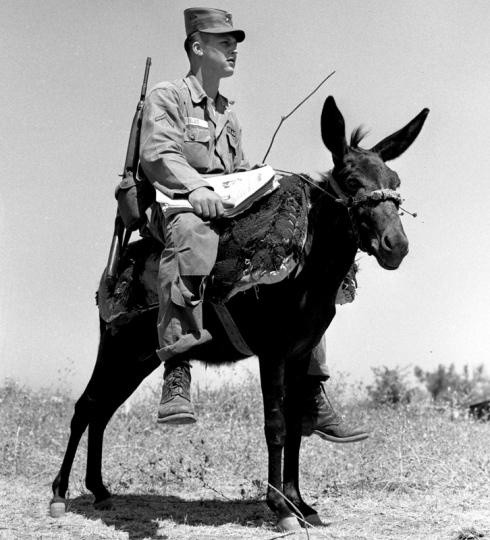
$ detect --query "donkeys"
[49,96,430,532]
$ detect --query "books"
[156,165,281,219]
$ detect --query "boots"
[302,383,369,443]
[157,359,197,424]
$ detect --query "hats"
[184,7,245,42]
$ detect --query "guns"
[106,57,152,290]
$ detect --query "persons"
[137,8,368,443]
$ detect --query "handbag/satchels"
[114,176,145,231]
